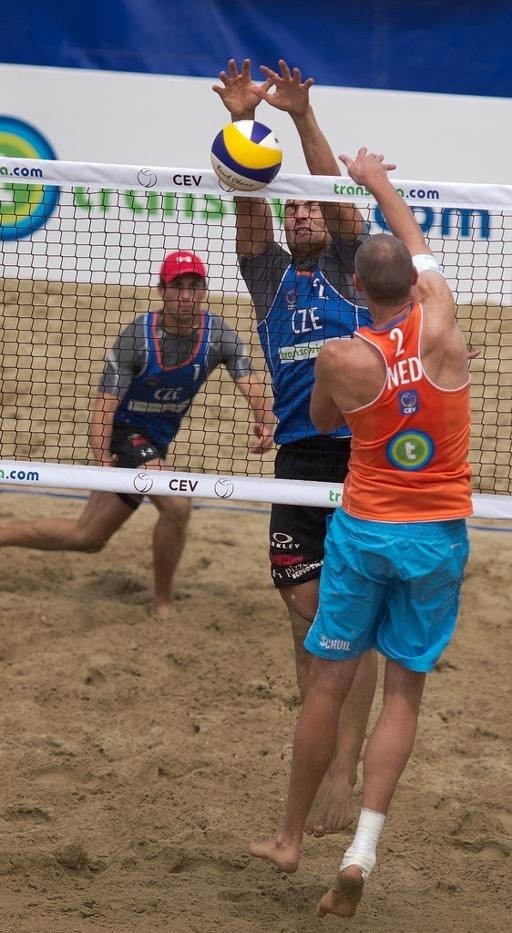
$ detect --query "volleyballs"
[211,120,282,191]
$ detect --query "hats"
[160,251,206,285]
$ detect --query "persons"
[210,54,380,838]
[0,245,278,619]
[246,141,476,922]
